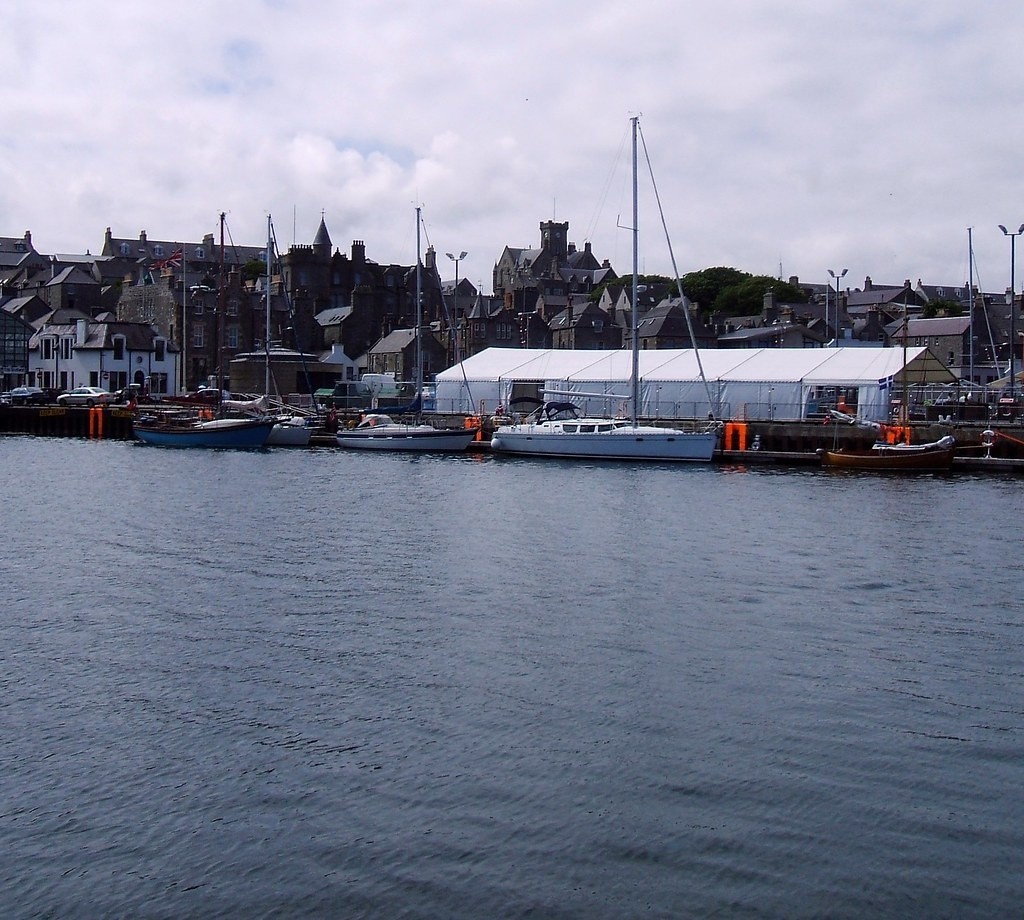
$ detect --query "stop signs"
[103,374,109,380]
[37,373,42,378]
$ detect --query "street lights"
[518,310,539,349]
[998,224,1024,400]
[446,252,468,367]
[827,269,848,348]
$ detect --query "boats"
[821,292,955,469]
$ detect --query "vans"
[362,374,396,390]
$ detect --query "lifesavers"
[198,410,212,419]
[979,429,996,447]
[988,404,997,416]
[1000,404,1011,416]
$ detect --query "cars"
[192,388,230,401]
[56,387,116,407]
[43,388,65,405]
[2,387,49,406]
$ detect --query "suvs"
[416,387,436,402]
[331,381,372,409]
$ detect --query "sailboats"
[491,115,720,462]
[131,212,291,449]
[925,228,1024,421]
[264,214,313,447]
[335,207,480,451]
